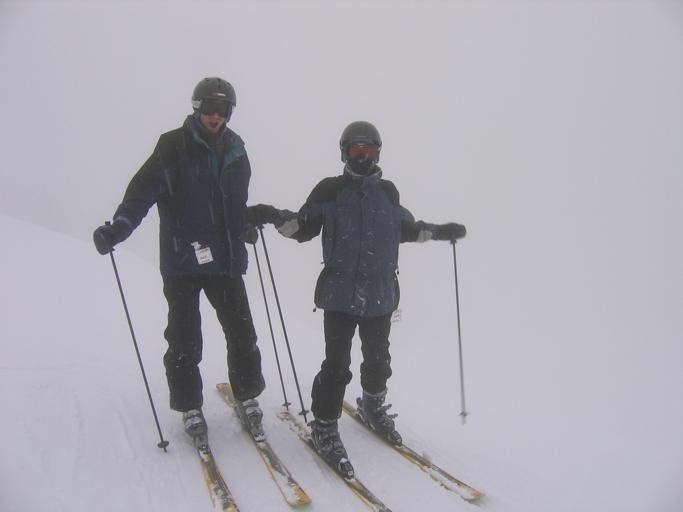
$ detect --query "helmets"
[339,120,382,164]
[192,76,237,123]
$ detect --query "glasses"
[199,99,230,119]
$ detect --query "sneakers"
[237,400,264,423]
[180,411,209,438]
[361,391,397,434]
[312,417,349,461]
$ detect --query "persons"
[91,75,266,438]
[244,120,467,466]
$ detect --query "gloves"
[93,219,131,256]
[247,202,279,231]
[436,223,467,242]
